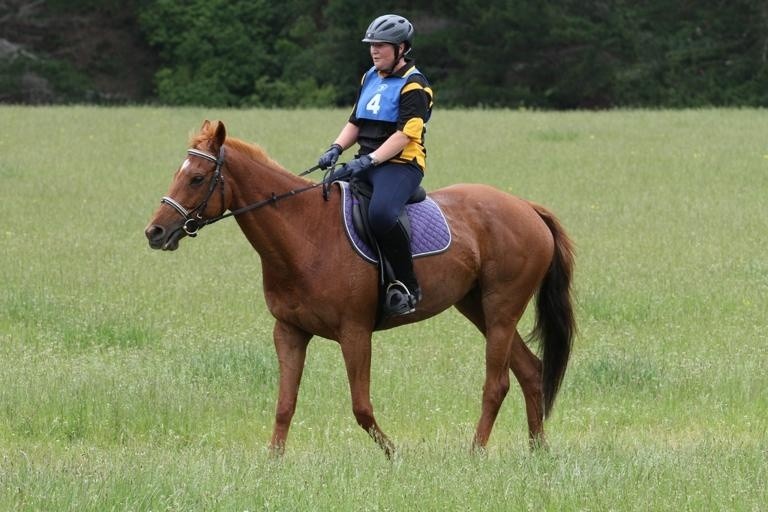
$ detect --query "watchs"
[369,154,378,166]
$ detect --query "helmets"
[361,14,414,47]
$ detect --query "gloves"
[318,144,342,170]
[343,155,373,177]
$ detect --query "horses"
[143,117,584,465]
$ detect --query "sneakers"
[398,285,422,316]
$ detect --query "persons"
[316,14,432,314]
